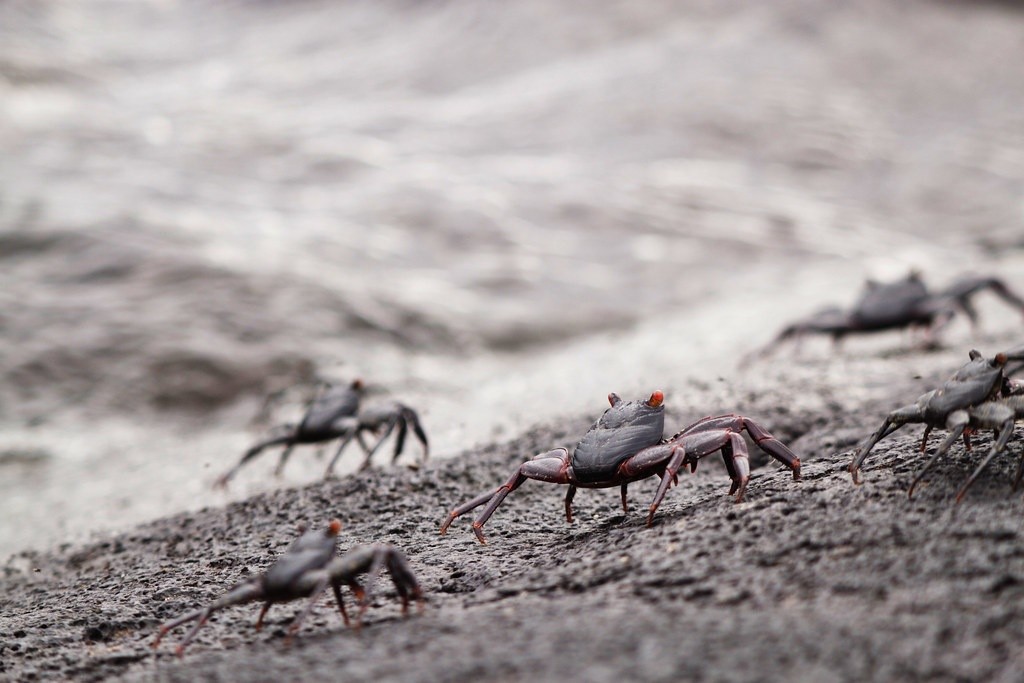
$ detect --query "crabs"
[777,269,1024,351]
[210,380,430,490]
[850,350,1024,503]
[146,520,423,657]
[438,392,801,546]
